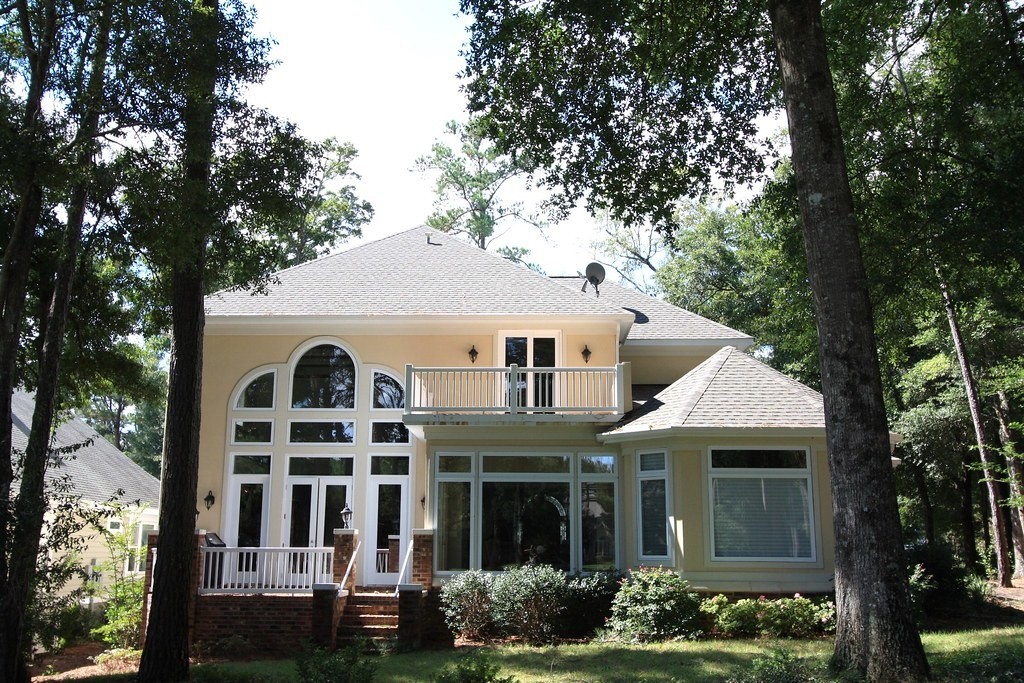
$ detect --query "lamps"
[582,345,592,364]
[421,497,425,511]
[469,344,479,363]
[340,503,352,529]
[204,491,214,511]
